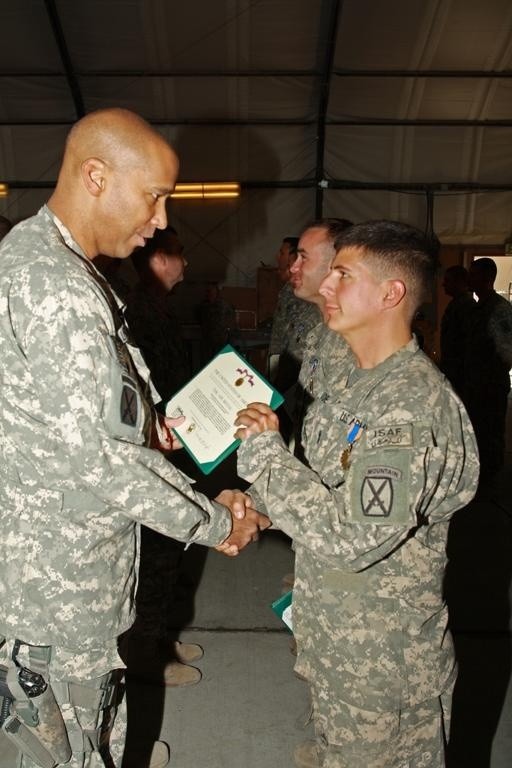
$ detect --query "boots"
[145,740,170,768]
[294,738,328,767]
[158,659,202,687]
[156,618,204,662]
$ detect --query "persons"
[207,218,482,767]
[0,107,277,767]
[197,233,324,397]
[437,263,476,372]
[278,216,361,451]
[109,224,204,688]
[461,257,512,487]
